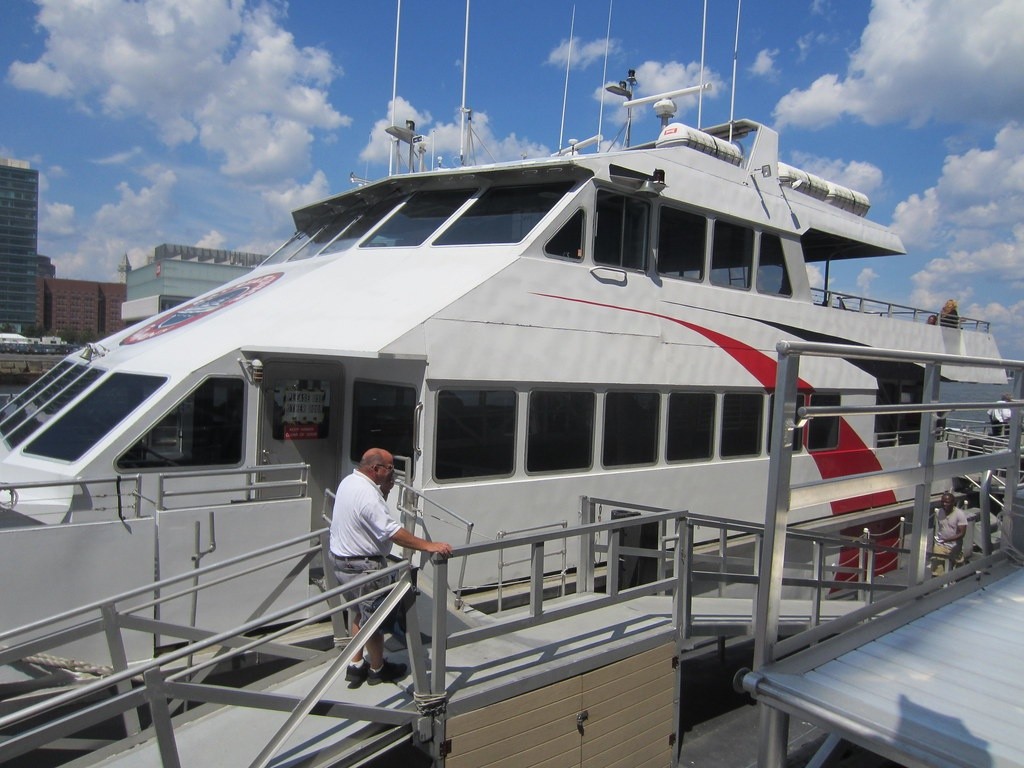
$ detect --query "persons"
[327,447,453,687]
[986,393,1014,437]
[940,299,958,329]
[931,491,968,590]
[927,315,937,325]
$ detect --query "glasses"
[372,463,394,471]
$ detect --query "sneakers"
[368,658,407,685]
[345,659,370,683]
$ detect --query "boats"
[0,0,1009,696]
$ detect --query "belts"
[329,550,384,561]
